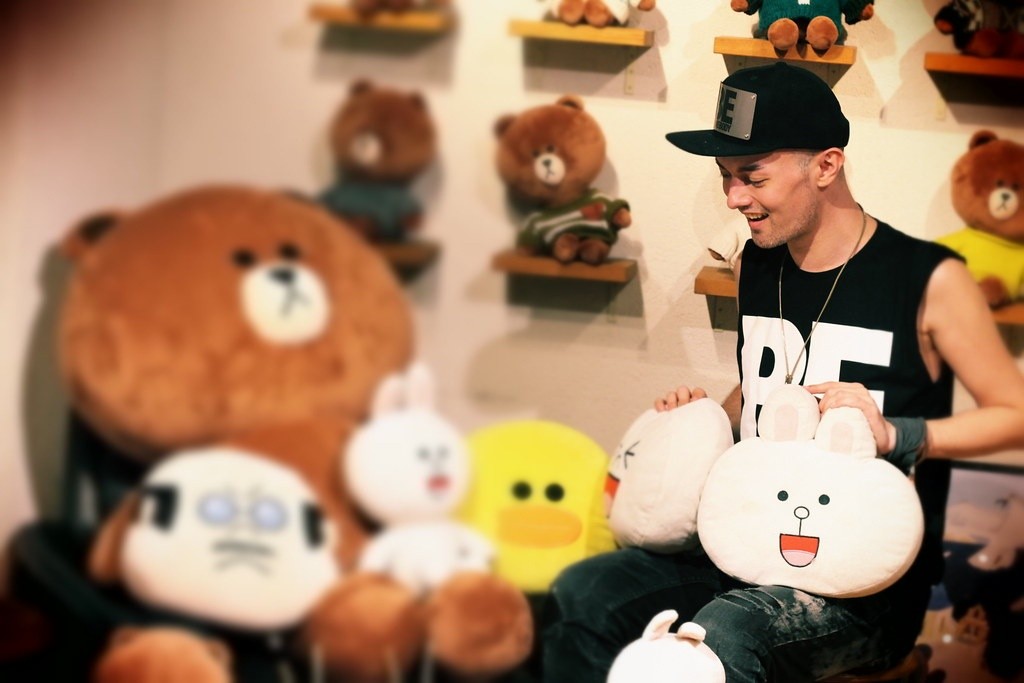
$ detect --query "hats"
[665,61,850,157]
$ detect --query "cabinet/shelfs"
[309,3,1024,329]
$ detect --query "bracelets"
[884,416,925,469]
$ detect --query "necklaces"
[779,200,866,383]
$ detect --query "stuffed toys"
[730,0,876,52]
[940,131,1024,303]
[458,418,617,595]
[535,1,658,27]
[604,397,734,553]
[62,190,535,683]
[315,78,433,248]
[934,0,1024,56]
[696,384,925,598]
[495,93,632,263]
[606,609,726,683]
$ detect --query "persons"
[543,62,1024,683]
